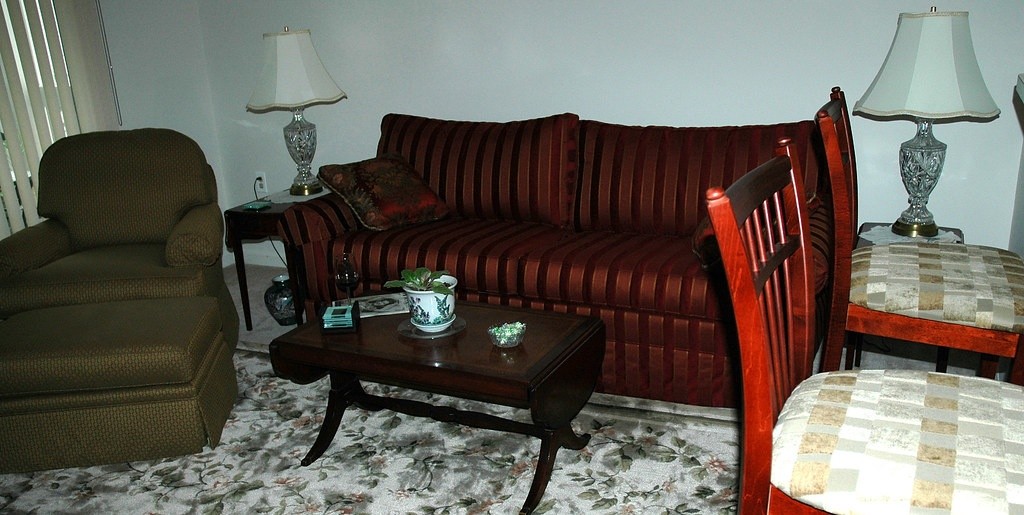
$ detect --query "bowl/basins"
[488,323,526,347]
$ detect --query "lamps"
[853,6,1000,237]
[245,25,347,196]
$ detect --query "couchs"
[278,112,832,409]
[0,127,240,478]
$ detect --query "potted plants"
[383,267,468,333]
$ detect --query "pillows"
[712,194,824,267]
[316,151,451,231]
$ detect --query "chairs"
[814,85,1024,384]
[706,138,1024,515]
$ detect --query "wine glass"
[334,253,360,308]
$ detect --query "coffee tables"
[268,301,605,515]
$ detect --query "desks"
[224,187,332,331]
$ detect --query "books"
[333,292,410,319]
